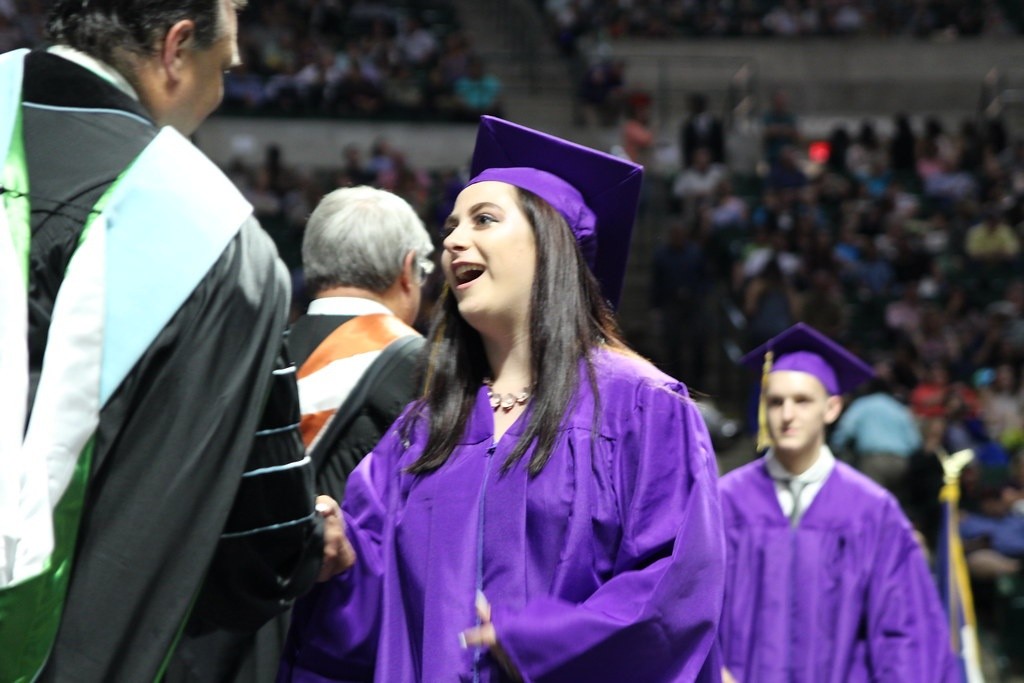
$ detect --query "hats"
[742,323,870,451]
[462,114,643,313]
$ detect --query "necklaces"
[481,374,537,409]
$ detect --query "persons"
[0,0,1024,683]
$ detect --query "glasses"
[408,255,436,286]
